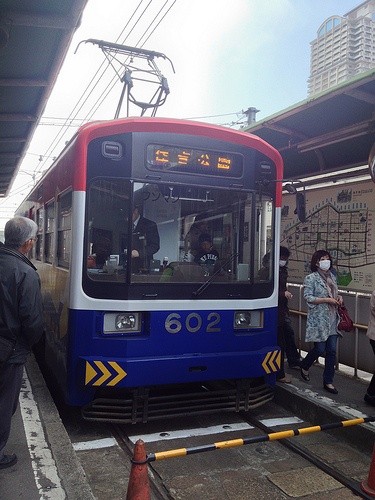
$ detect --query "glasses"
[25,236,38,244]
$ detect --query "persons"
[0,215,43,468]
[87,198,161,273]
[300,251,344,395]
[364,288,375,407]
[183,215,220,266]
[255,245,301,383]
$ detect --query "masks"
[319,260,330,271]
[280,260,287,267]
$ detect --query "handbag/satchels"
[337,300,353,332]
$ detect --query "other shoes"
[0,453,17,469]
[301,367,310,381]
[279,378,291,383]
[323,383,339,394]
[363,394,375,406]
[291,365,301,369]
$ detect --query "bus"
[12,117,308,424]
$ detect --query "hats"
[198,234,214,242]
[118,200,140,214]
